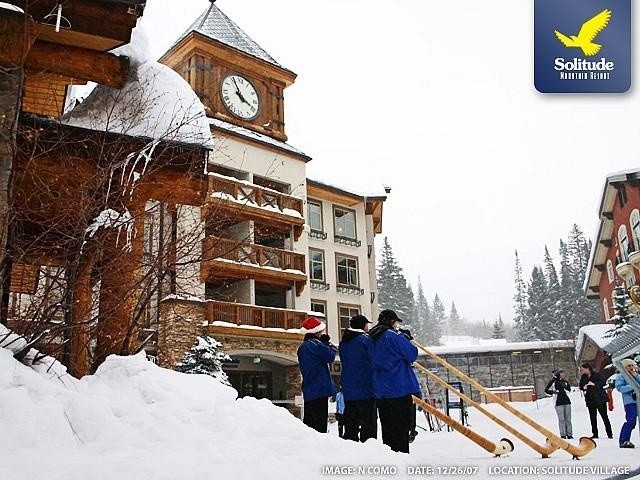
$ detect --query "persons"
[614,358,640,449]
[370,309,418,453]
[327,413,339,434]
[579,363,614,439]
[296,316,337,433]
[336,385,346,437]
[544,366,575,440]
[338,314,377,442]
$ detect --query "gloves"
[399,324,416,340]
[321,334,330,342]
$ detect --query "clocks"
[220,72,262,122]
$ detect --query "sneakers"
[620,440,635,448]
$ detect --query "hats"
[350,315,373,328]
[623,359,636,368]
[300,316,326,335]
[378,309,402,322]
